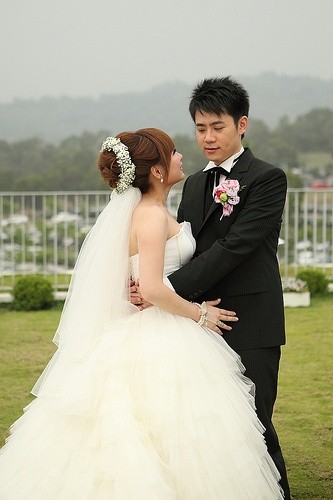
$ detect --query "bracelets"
[194,301,208,327]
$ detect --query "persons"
[126,75,291,500]
[97,128,240,500]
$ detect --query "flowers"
[213,180,241,221]
